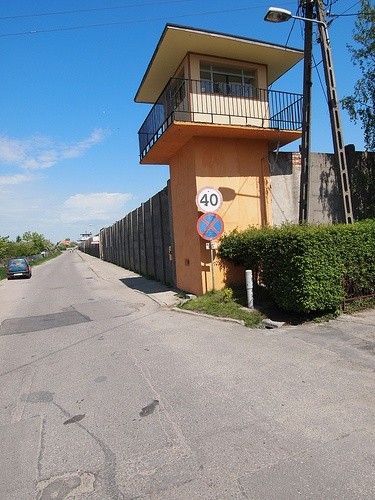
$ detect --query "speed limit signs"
[195,186,224,214]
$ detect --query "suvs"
[7,257,32,278]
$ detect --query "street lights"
[264,6,356,224]
